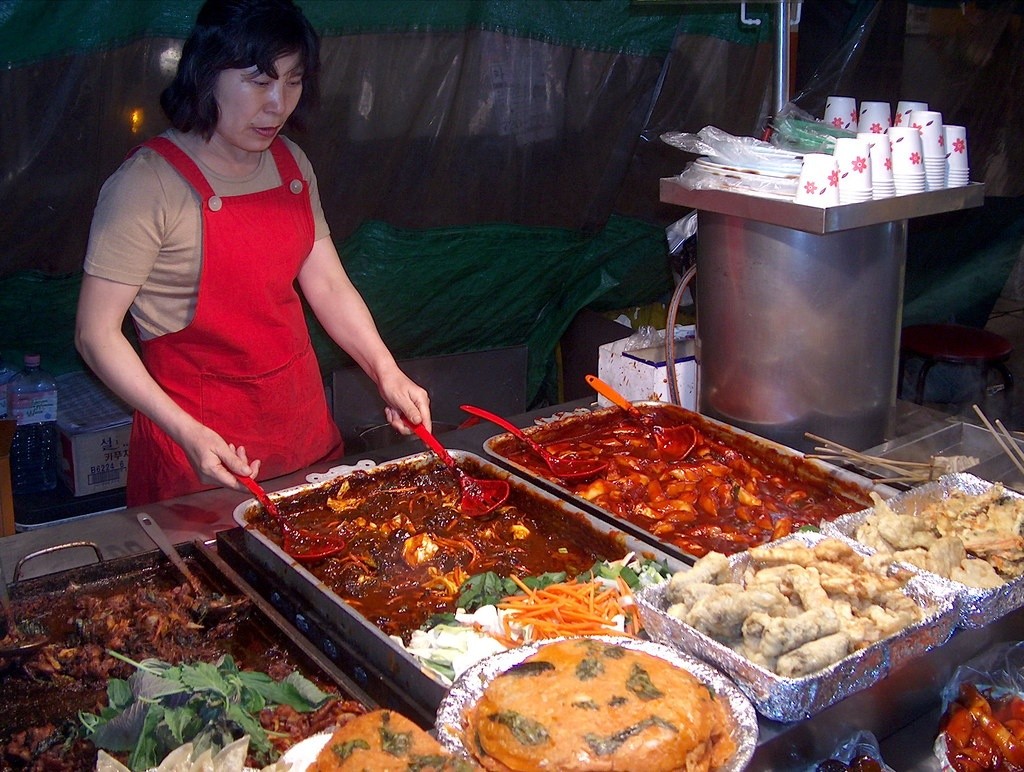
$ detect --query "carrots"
[474,568,643,647]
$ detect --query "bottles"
[8,354,59,493]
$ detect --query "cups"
[941,125,969,188]
[823,96,857,135]
[857,102,892,134]
[857,133,895,201]
[893,101,928,128]
[908,110,946,192]
[887,126,925,197]
[833,137,874,205]
[791,153,841,209]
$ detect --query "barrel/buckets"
[695,208,908,463]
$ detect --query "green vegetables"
[78,651,338,766]
[424,560,672,626]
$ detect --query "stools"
[896,320,1014,414]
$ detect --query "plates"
[691,147,804,196]
[778,117,857,154]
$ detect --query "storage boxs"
[599,322,696,410]
[50,370,135,495]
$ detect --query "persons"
[74,0,434,509]
